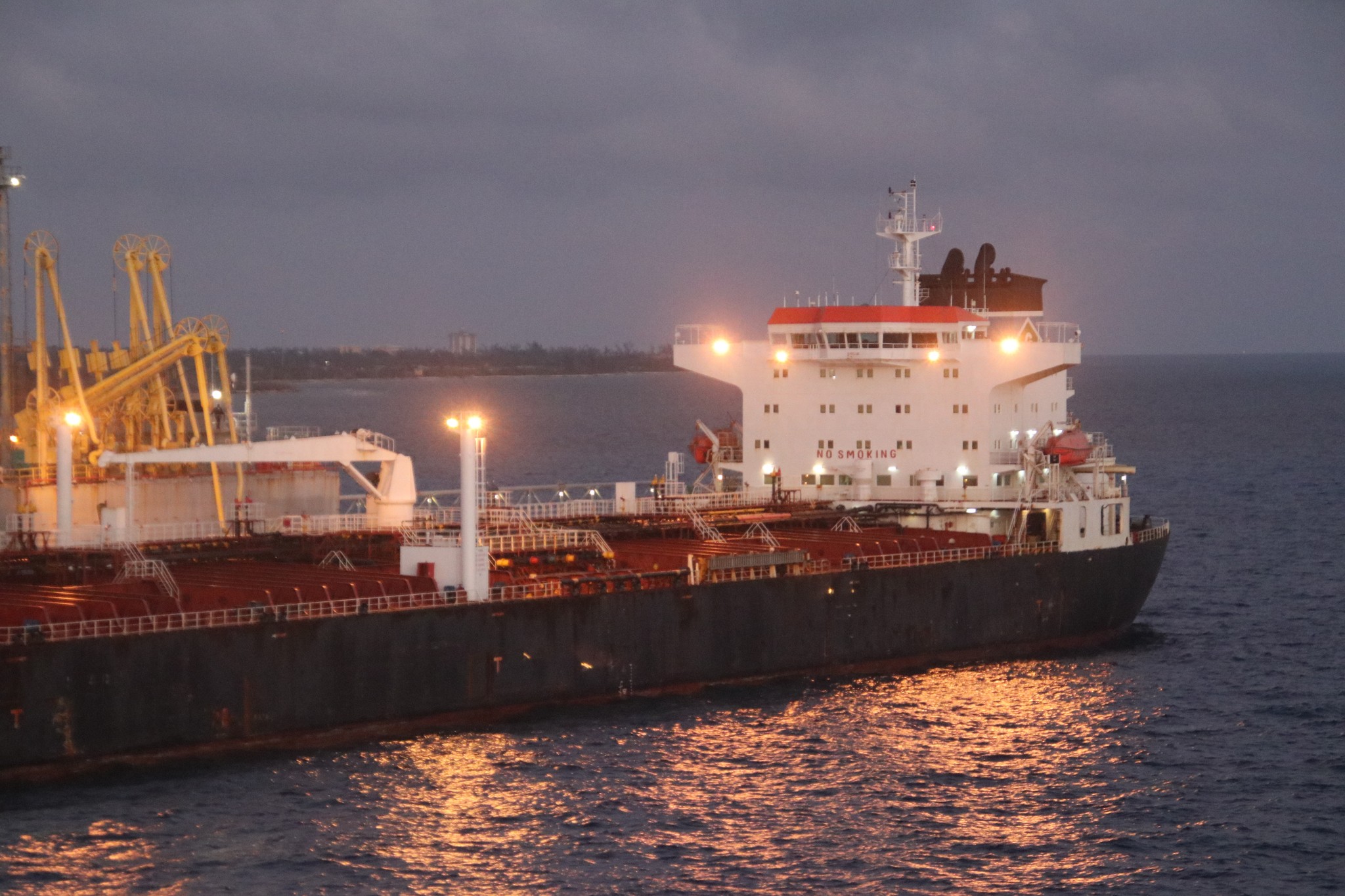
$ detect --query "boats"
[1040,421,1095,468]
[688,427,742,465]
[0,173,1173,799]
